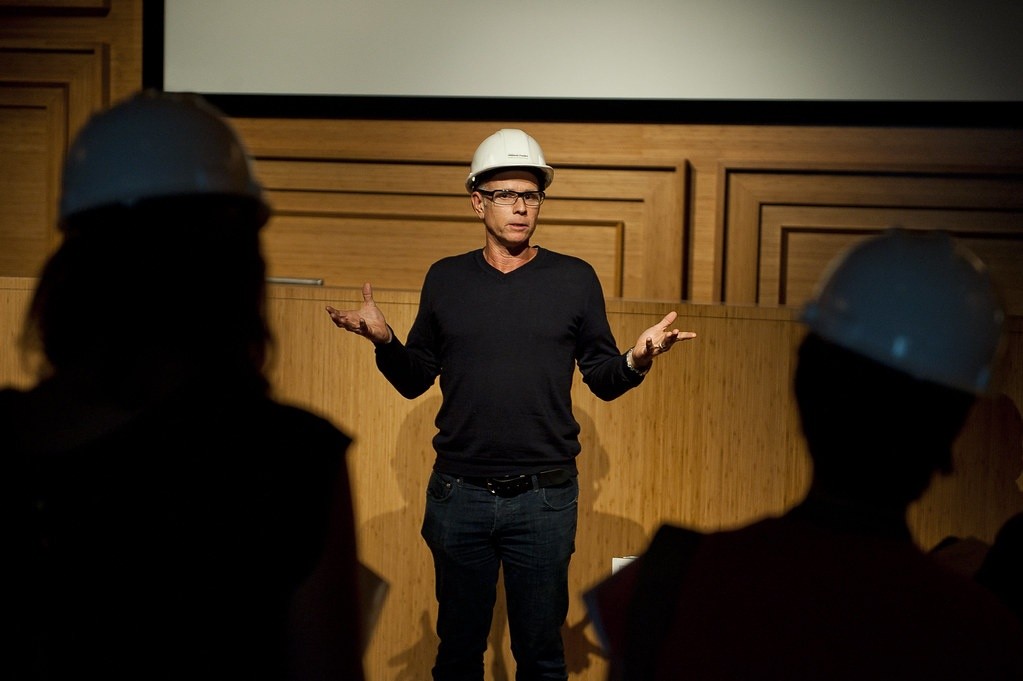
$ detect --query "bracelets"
[626,349,650,376]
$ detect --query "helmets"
[465,128,555,193]
[52,90,274,229]
[795,228,1000,399]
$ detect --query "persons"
[324,129,697,681]
[582,229,1023,681]
[0,96,369,681]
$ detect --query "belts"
[462,463,579,495]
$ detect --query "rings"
[658,345,667,353]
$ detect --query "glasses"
[478,190,546,207]
